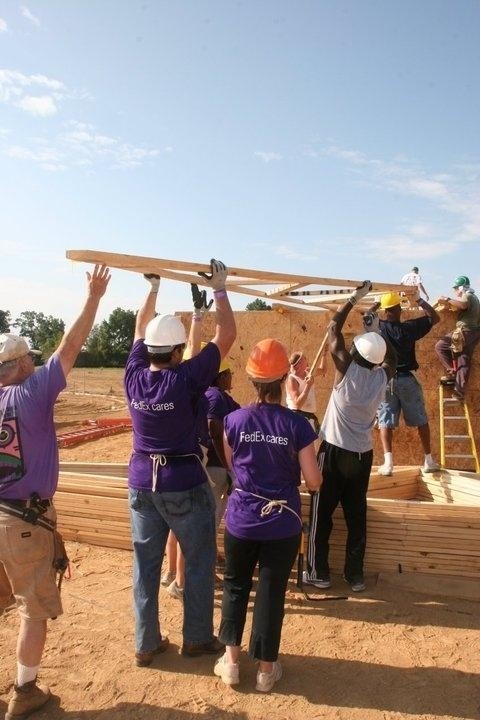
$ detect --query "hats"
[290,350,303,368]
[0,332,42,363]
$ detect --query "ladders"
[56,423,126,446]
[66,250,418,312]
[439,383,480,473]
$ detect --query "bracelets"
[444,297,453,305]
[416,300,426,306]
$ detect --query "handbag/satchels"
[50,526,70,575]
[450,327,465,354]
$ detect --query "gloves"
[412,289,424,306]
[190,283,215,325]
[363,312,382,334]
[348,279,373,307]
[143,272,162,294]
[198,258,229,299]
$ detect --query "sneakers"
[299,571,333,589]
[424,466,440,473]
[440,375,455,380]
[378,468,393,476]
[213,656,240,685]
[133,636,169,667]
[181,637,225,657]
[450,392,464,399]
[342,575,365,592]
[166,581,185,603]
[255,661,283,694]
[160,568,177,586]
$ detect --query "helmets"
[181,341,230,374]
[143,313,188,353]
[246,338,291,383]
[452,275,470,288]
[381,292,402,308]
[352,332,388,365]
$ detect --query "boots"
[5,678,52,720]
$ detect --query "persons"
[358,292,444,480]
[1,260,116,720]
[214,338,325,691]
[282,336,335,437]
[398,263,433,306]
[433,273,480,404]
[122,258,242,667]
[297,279,390,596]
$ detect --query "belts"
[1,498,53,510]
[393,368,411,374]
